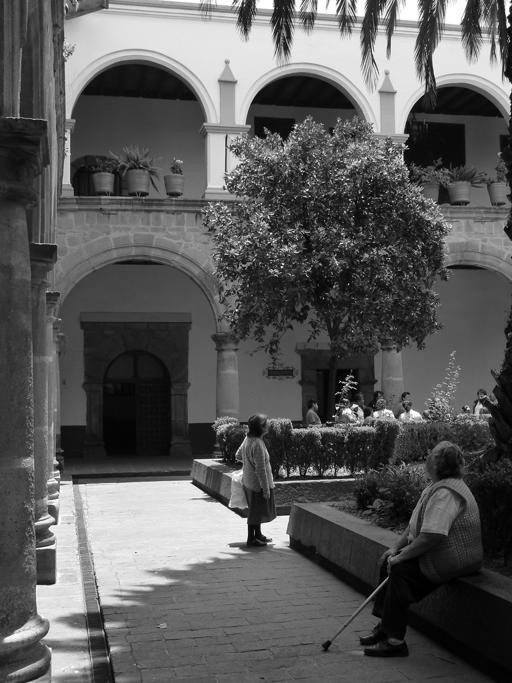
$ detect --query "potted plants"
[90,143,186,196]
[412,151,509,205]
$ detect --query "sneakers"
[357,622,387,645]
[362,635,409,658]
[246,533,273,547]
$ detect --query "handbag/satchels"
[228,472,249,510]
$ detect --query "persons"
[303,387,424,426]
[231,410,280,547]
[472,388,492,414]
[358,439,489,660]
[463,404,472,413]
